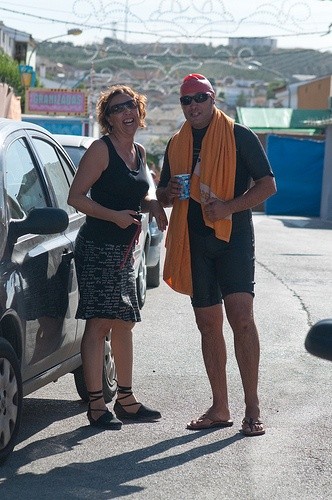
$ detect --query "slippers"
[241,417,264,435]
[186,414,234,430]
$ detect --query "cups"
[174,174,192,201]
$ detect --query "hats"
[180,73,214,100]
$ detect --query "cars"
[0,116,147,462]
[52,132,161,287]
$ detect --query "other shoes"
[113,399,162,422]
[87,403,123,429]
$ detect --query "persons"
[67,86,169,429]
[156,72,277,437]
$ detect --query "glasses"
[108,100,138,114]
[180,91,215,105]
